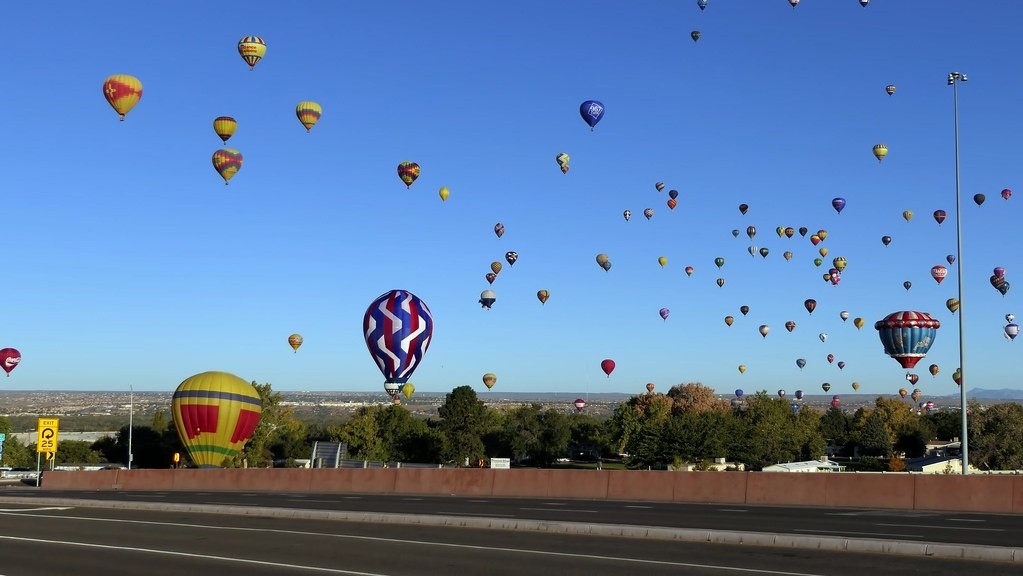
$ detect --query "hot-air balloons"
[480,289,497,309]
[288,333,304,353]
[603,261,613,270]
[485,272,498,284]
[600,359,616,377]
[884,84,896,98]
[361,288,437,407]
[402,383,414,399]
[236,34,266,73]
[102,74,142,125]
[210,147,243,185]
[621,144,1019,430]
[505,251,519,267]
[494,223,506,240]
[0,347,21,378]
[597,253,608,266]
[167,369,262,469]
[395,161,422,188]
[789,0,799,8]
[859,0,870,8]
[691,31,700,43]
[573,398,586,412]
[580,99,605,132]
[491,262,502,274]
[556,151,571,168]
[537,289,550,304]
[560,164,571,174]
[293,101,324,134]
[696,0,708,10]
[482,372,497,391]
[437,187,448,203]
[213,117,238,146]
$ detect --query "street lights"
[944,72,971,479]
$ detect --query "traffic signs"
[37,418,59,454]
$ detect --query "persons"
[595,454,603,470]
